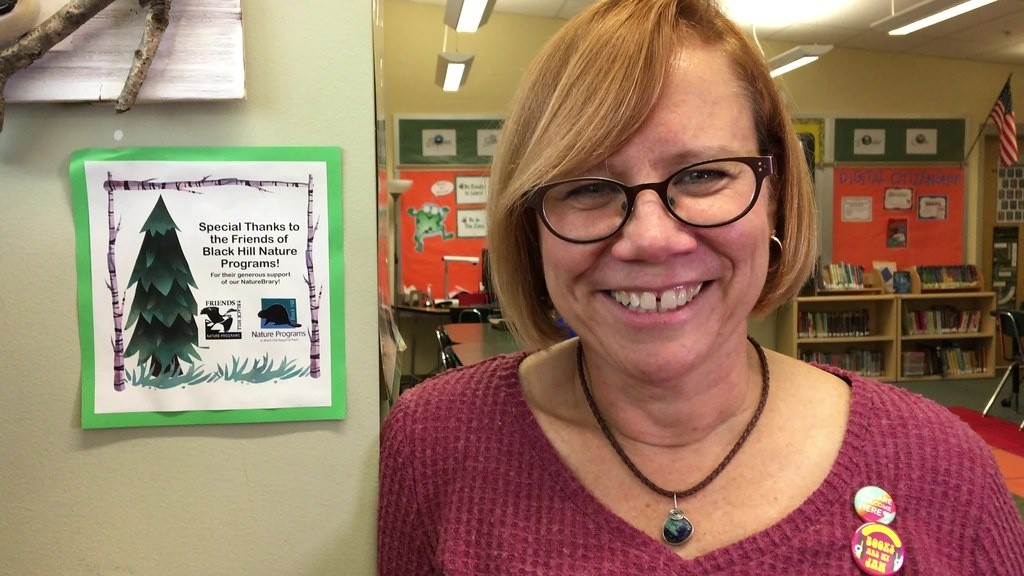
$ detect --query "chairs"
[983,307,1024,430]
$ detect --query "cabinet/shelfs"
[774,270,899,384]
[898,265,997,382]
[982,223,1024,370]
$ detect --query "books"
[798,227,1020,377]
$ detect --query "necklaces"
[576,327,770,544]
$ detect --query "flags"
[990,77,1020,170]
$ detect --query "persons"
[377,0,1024,576]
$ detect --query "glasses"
[517,153,778,242]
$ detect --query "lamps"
[752,30,834,80]
[869,0,999,36]
[444,0,495,33]
[435,24,473,92]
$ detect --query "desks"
[436,321,522,370]
[390,302,503,388]
